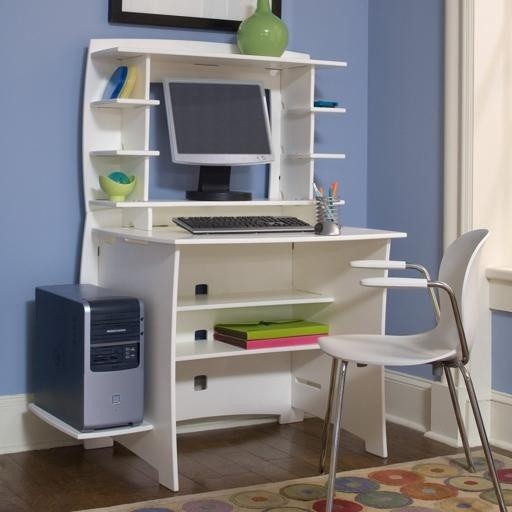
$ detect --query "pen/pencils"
[313,182,337,220]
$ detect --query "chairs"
[317,225,508,512]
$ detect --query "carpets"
[90,439,512,512]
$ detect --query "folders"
[214,320,328,350]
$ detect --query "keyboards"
[172,216,315,234]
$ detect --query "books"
[212,333,329,350]
[214,319,329,341]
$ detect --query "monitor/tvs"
[162,77,277,201]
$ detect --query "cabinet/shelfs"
[84,38,349,228]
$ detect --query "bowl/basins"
[99,175,137,202]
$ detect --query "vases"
[235,1,289,57]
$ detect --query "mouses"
[315,221,341,236]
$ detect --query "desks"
[28,226,406,491]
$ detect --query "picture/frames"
[107,0,283,30]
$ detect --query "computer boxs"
[35,284,145,433]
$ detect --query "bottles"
[316,197,342,235]
